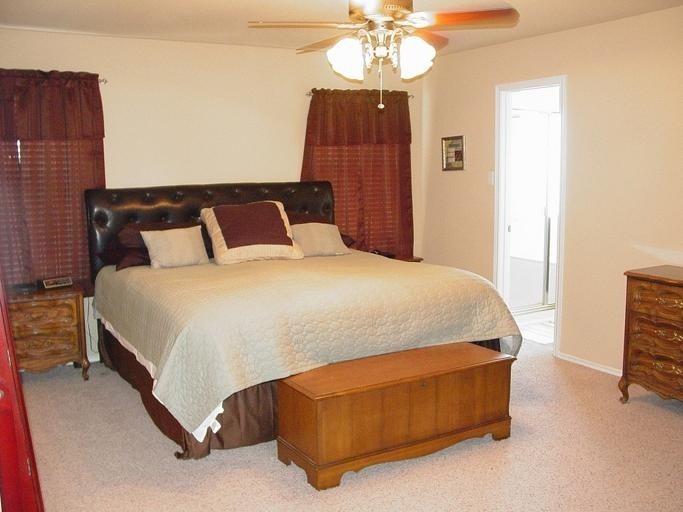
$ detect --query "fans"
[245,1,525,53]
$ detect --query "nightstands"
[5,283,91,384]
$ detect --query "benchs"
[274,342,519,490]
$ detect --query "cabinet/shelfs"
[617,263,683,407]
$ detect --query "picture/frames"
[439,134,466,172]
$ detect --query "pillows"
[110,195,358,271]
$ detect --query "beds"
[81,180,514,455]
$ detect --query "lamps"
[326,32,436,109]
